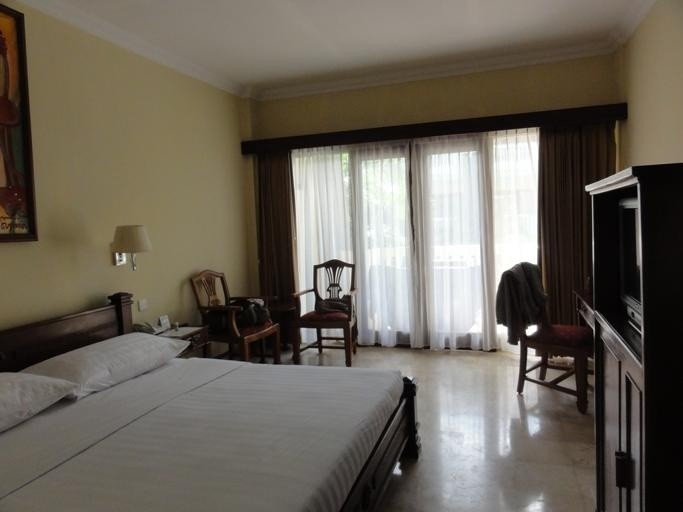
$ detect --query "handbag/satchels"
[220,298,270,338]
[314,296,348,314]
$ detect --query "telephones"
[133,321,165,335]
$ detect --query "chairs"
[505,263,594,414]
[189,259,359,367]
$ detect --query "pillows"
[1,329,193,436]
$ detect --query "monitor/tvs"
[619,197,642,336]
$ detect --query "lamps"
[110,224,152,272]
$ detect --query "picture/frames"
[0,3,39,243]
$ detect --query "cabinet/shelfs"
[581,161,683,511]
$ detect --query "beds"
[0,289,422,512]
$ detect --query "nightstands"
[157,324,210,358]
